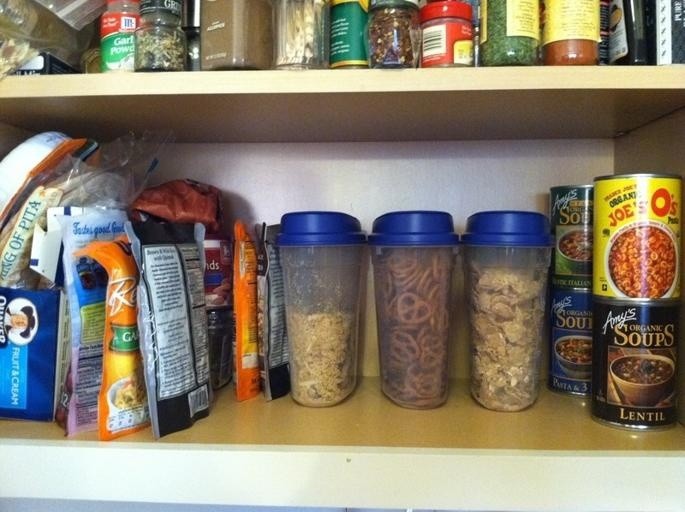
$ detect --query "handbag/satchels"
[130,179,225,233]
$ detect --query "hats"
[20,306,35,337]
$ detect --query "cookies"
[374,248,453,407]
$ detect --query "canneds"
[329,0,601,69]
[99,0,142,73]
[546,172,684,430]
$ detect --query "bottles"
[460,209,555,411]
[273,210,369,407]
[133,0,188,72]
[270,0,332,69]
[199,0,274,71]
[368,210,460,411]
[364,0,423,69]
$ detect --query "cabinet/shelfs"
[0,66,684,509]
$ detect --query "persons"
[4,303,37,338]
